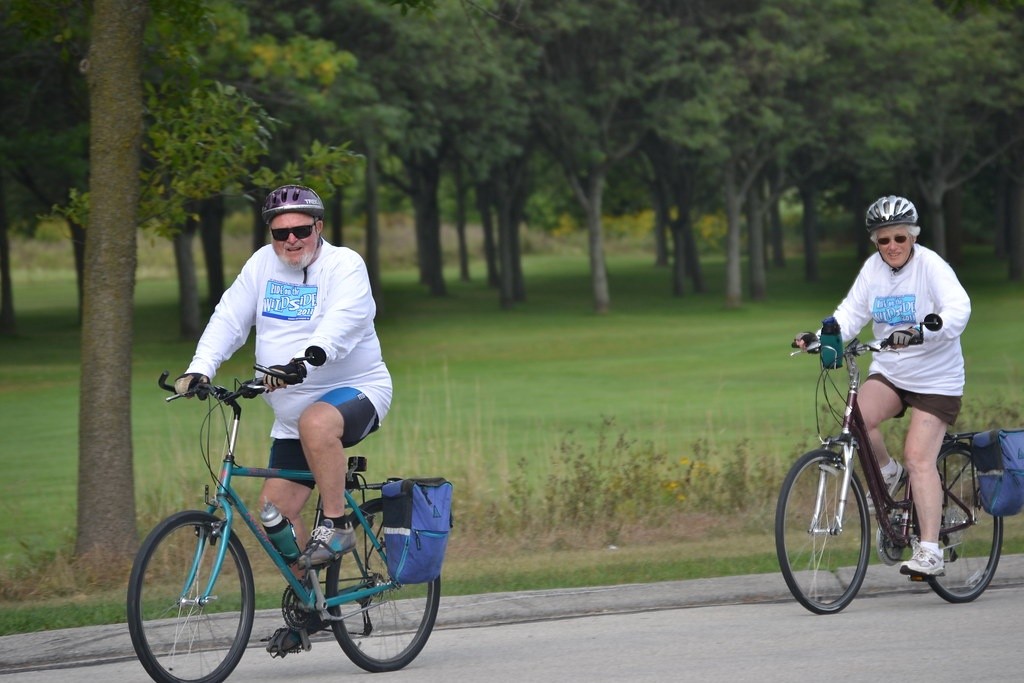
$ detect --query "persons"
[794,203,972,576]
[175,191,392,652]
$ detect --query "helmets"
[865,195,919,234]
[262,185,325,226]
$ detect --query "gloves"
[173,372,210,401]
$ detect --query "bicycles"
[124,362,457,683]
[772,314,1024,615]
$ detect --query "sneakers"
[865,458,909,516]
[296,522,358,570]
[899,540,945,576]
[266,604,332,653]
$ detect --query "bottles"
[819,316,844,370]
[257,495,302,565]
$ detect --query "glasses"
[877,235,909,245]
[266,218,320,242]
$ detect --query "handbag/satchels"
[380,477,455,585]
[970,427,1023,517]
